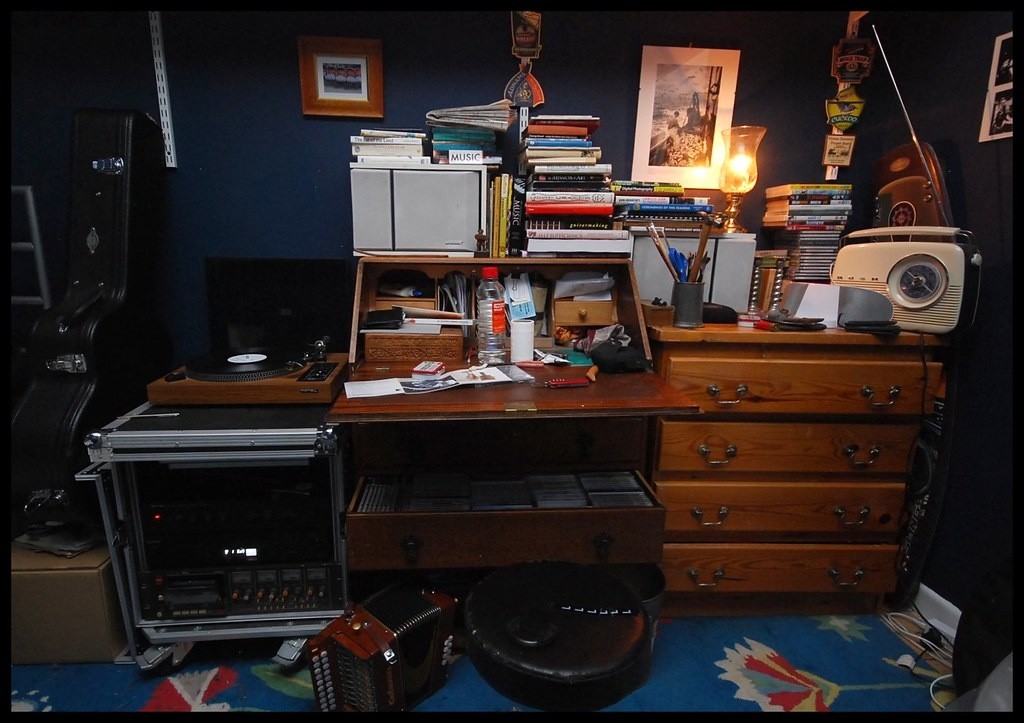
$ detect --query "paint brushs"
[645,218,681,283]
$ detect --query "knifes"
[486,361,547,368]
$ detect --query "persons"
[323,66,361,89]
[665,111,681,147]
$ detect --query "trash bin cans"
[594,561,667,659]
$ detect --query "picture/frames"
[629,43,740,189]
[298,35,385,118]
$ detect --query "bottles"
[476,266,506,365]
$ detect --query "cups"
[671,280,706,328]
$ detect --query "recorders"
[831,24,982,335]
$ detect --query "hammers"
[688,210,723,283]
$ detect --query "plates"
[762,317,824,326]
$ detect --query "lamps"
[710,125,768,232]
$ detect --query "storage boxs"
[411,359,445,380]
[365,326,465,360]
[11,535,127,666]
[640,299,675,329]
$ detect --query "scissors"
[669,248,688,283]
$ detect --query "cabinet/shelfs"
[646,321,954,615]
[325,359,705,570]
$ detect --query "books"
[357,471,653,514]
[753,185,852,319]
[349,100,512,167]
[488,115,728,259]
